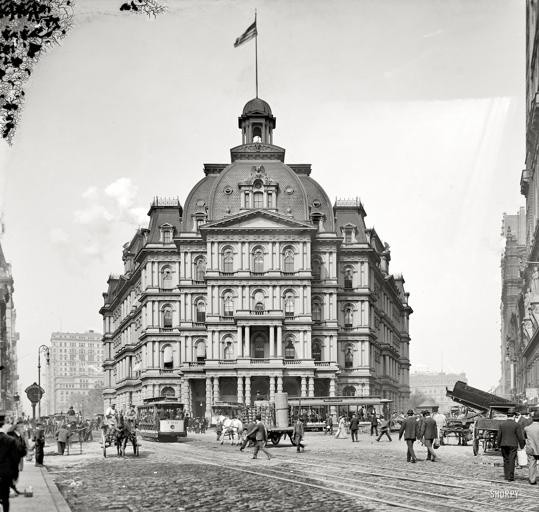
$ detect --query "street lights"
[36,344,50,440]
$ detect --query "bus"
[254,395,394,435]
[134,398,187,444]
[210,403,248,428]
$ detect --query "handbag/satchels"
[532,411,539,419]
[434,438,440,448]
[505,409,517,415]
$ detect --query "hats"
[423,410,430,416]
[407,409,414,414]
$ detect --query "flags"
[233,20,257,49]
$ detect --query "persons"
[419,410,440,461]
[289,407,447,448]
[214,412,272,460]
[124,405,139,427]
[0,412,21,511]
[497,408,525,482]
[513,408,533,469]
[399,408,420,462]
[140,409,210,435]
[293,417,305,453]
[524,411,539,484]
[1,404,92,475]
[104,403,118,430]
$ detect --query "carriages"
[215,406,304,448]
[53,409,94,445]
[98,406,140,459]
